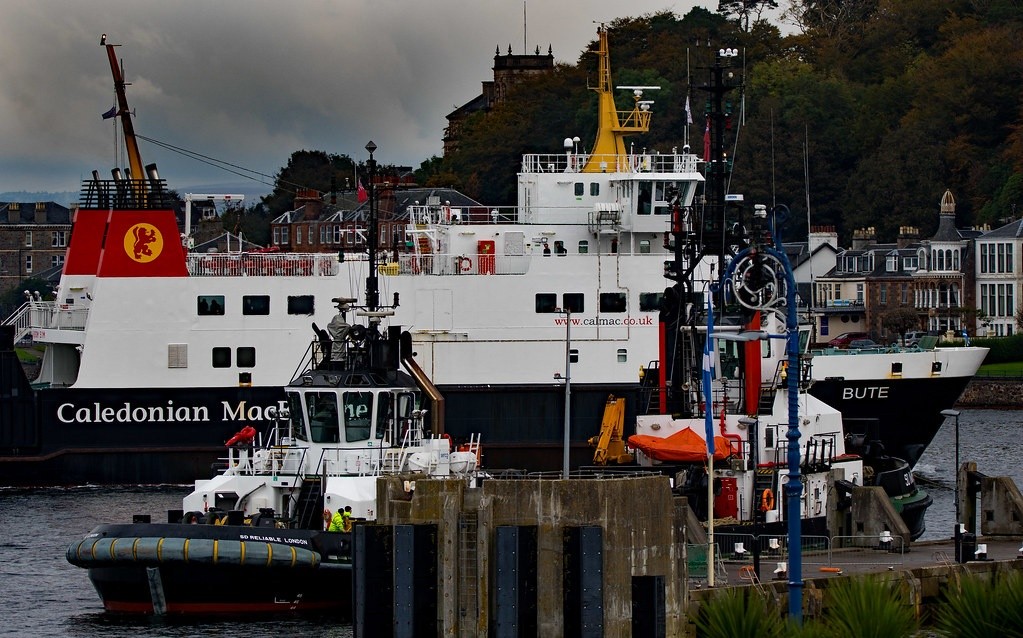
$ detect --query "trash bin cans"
[955,534,976,562]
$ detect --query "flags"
[358,179,368,203]
[684,96,693,123]
[704,119,710,161]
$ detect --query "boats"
[66,140,494,623]
[1,19,990,558]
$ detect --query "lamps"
[302,375,315,385]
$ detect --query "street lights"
[939,408,960,563]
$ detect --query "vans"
[898,332,927,348]
[829,331,868,348]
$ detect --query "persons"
[556,241,567,256]
[211,300,220,312]
[328,506,352,532]
[200,298,208,314]
[543,243,551,256]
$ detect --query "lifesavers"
[444,434,452,452]
[762,488,774,510]
[461,257,472,271]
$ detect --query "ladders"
[466,433,481,474]
[428,433,441,475]
